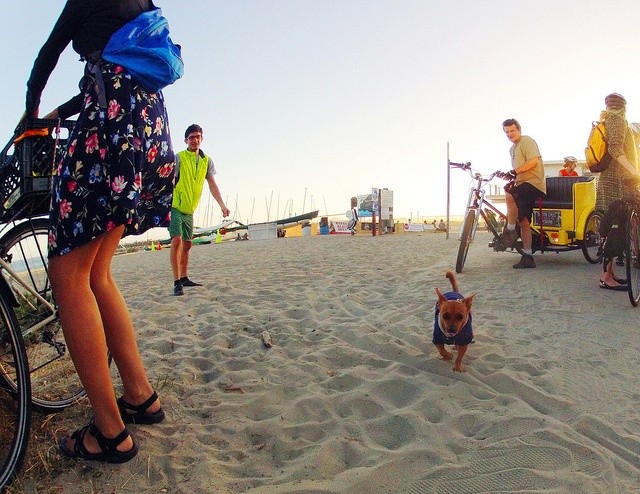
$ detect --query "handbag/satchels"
[101,0,183,94]
[597,199,628,272]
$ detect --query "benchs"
[404,223,424,233]
[529,176,597,229]
[423,223,435,233]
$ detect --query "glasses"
[185,135,202,140]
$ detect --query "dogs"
[431,271,477,374]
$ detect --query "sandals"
[60,423,137,463]
[90,391,165,424]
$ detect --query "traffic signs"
[151,240,155,251]
[216,228,221,243]
[156,241,161,249]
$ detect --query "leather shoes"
[599,277,627,290]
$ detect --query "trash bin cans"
[301,222,311,236]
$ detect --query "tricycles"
[448,161,608,273]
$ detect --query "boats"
[159,187,319,245]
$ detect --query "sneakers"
[174,284,183,295]
[183,279,202,286]
[494,228,518,252]
[513,253,536,268]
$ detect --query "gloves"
[505,171,517,180]
[504,181,514,192]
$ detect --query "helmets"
[565,157,577,163]
[605,92,627,104]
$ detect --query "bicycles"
[0,192,113,416]
[621,175,640,306]
[0,294,33,494]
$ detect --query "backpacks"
[585,115,612,172]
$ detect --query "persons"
[581,91,639,291]
[172,123,230,300]
[439,219,445,229]
[558,155,579,176]
[24,0,170,460]
[493,119,547,269]
